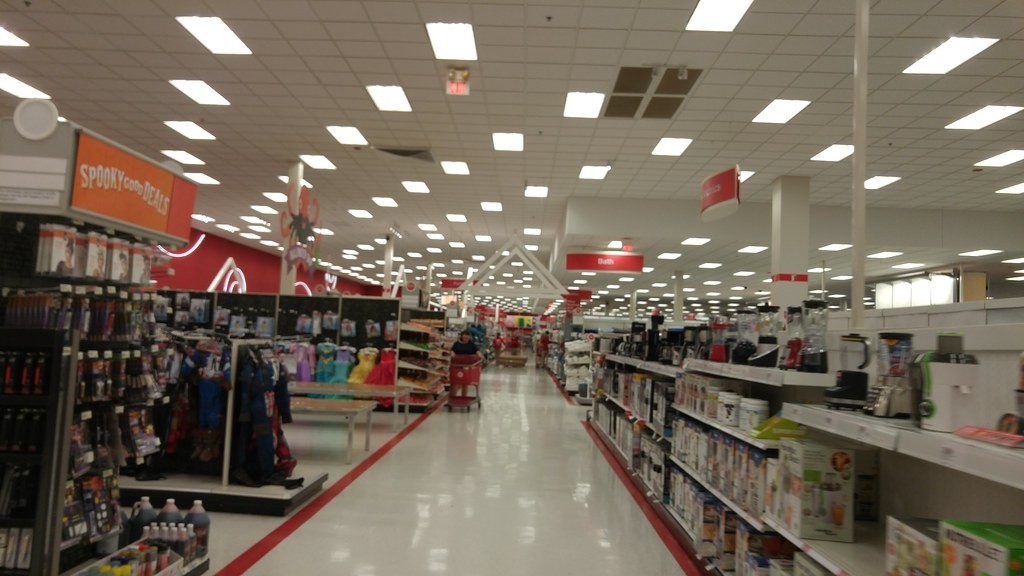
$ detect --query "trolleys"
[443,354,483,412]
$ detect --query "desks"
[289,396,377,463]
[285,381,410,432]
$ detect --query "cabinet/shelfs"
[563,339,594,401]
[591,350,679,503]
[399,322,448,415]
[0,222,209,576]
[758,324,1024,576]
[668,353,835,576]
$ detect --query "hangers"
[324,338,397,354]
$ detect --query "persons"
[509,331,521,354]
[92,248,106,277]
[492,331,506,366]
[443,330,484,407]
[55,238,76,274]
[218,308,271,340]
[342,321,397,340]
[156,293,205,326]
[540,330,557,367]
[138,255,151,283]
[535,339,541,368]
[117,250,129,282]
[301,316,337,330]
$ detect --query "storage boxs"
[593,372,1024,576]
